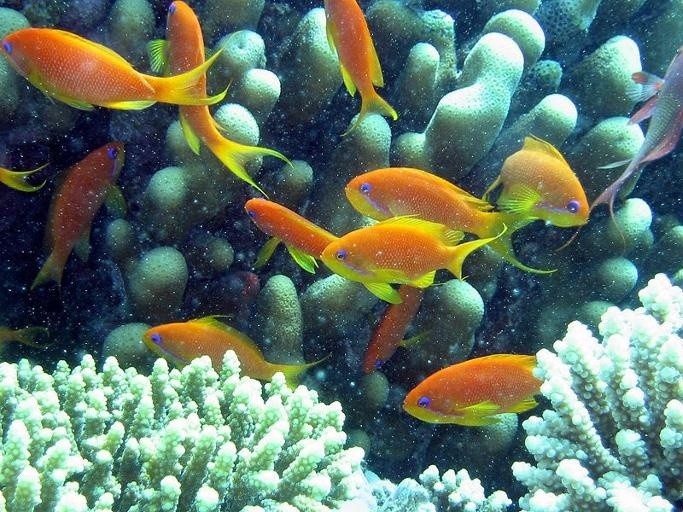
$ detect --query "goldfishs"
[357,271,431,374]
[242,133,591,303]
